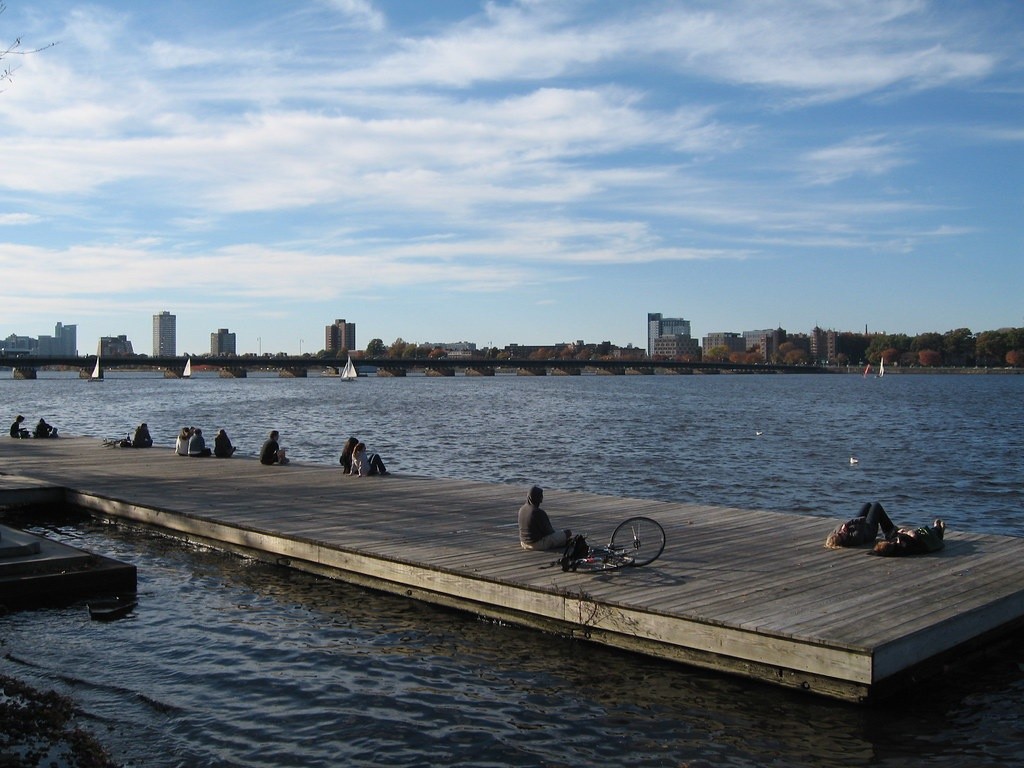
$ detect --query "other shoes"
[934,519,945,534]
[381,471,390,475]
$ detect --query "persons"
[347,443,391,477]
[132,423,153,447]
[188,430,211,456]
[35,419,52,438]
[876,519,945,556]
[826,501,899,549]
[175,427,194,455]
[259,431,289,465]
[10,415,26,438]
[340,437,360,474]
[518,488,571,550]
[214,430,235,458]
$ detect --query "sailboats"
[340,355,357,382]
[87,355,104,382]
[182,358,192,379]
[875,357,884,378]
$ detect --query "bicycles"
[556,515,667,574]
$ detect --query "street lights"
[257,336,262,357]
[300,339,304,355]
